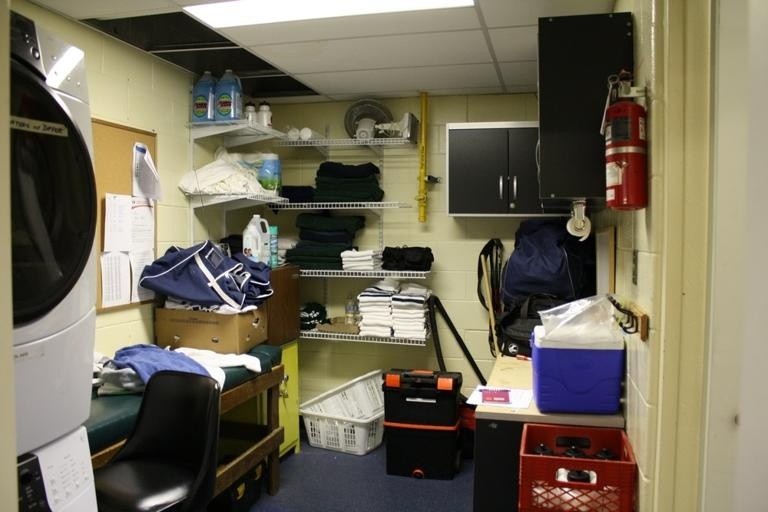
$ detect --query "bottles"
[345,296,355,325]
[256,100,272,129]
[244,100,256,121]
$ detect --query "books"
[482,390,513,405]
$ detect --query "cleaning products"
[243,224,260,262]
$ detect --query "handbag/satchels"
[139,240,273,309]
[490,216,596,356]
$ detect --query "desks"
[472,355,626,512]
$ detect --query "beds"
[80,344,284,512]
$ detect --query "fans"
[344,98,419,144]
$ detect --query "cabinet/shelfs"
[447,14,634,218]
[186,119,287,209]
[269,134,432,346]
[222,339,300,459]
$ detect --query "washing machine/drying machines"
[7,10,99,456]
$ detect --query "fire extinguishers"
[600,69,648,211]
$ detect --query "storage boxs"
[384,422,462,481]
[155,301,268,354]
[268,267,299,345]
[531,333,624,414]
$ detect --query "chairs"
[94,370,220,512]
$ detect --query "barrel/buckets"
[192,71,218,122]
[250,213,271,263]
[215,68,242,120]
